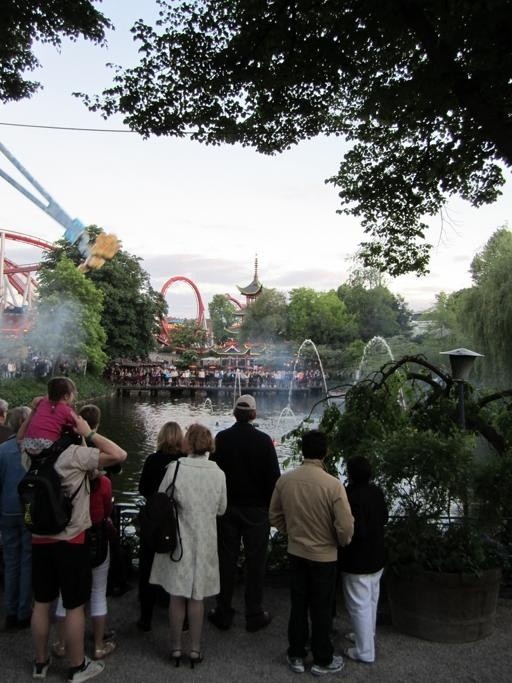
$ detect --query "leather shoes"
[245,611,272,633]
[208,608,231,630]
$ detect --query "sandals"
[52,640,117,659]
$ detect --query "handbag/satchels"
[265,548,293,581]
[86,522,108,568]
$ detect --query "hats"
[236,394,256,410]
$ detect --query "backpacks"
[17,446,83,536]
[136,461,184,562]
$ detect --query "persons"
[208,393,281,633]
[136,422,190,634]
[0,345,82,377]
[268,430,356,676]
[104,363,323,386]
[15,396,129,682]
[337,455,389,663]
[50,476,118,661]
[148,423,227,669]
[21,377,108,480]
[0,399,123,634]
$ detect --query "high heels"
[169,649,186,668]
[189,649,204,669]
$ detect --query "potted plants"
[304,366,512,644]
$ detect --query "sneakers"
[342,647,360,662]
[344,631,357,643]
[32,657,52,680]
[4,612,31,628]
[311,655,345,676]
[65,656,106,682]
[286,654,306,673]
[136,617,154,635]
